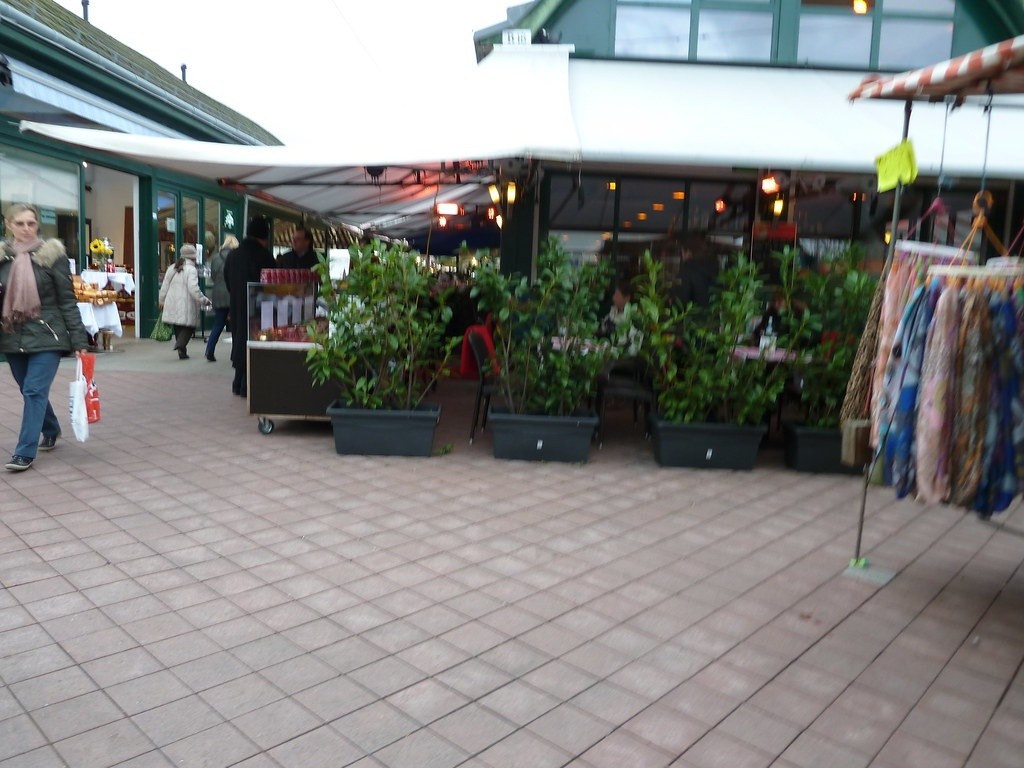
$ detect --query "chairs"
[459,325,501,446]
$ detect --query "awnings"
[18,116,518,237]
[9,71,162,137]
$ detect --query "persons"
[224,222,275,395]
[675,237,720,317]
[159,246,208,359]
[599,285,644,341]
[0,204,89,471]
[205,237,238,361]
[276,228,317,268]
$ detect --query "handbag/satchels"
[79,352,101,424]
[150,310,172,341]
[68,356,89,443]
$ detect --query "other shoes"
[205,351,216,361]
[173,343,188,352]
[179,353,189,359]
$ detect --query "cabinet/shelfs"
[192,276,219,343]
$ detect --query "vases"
[99,257,105,271]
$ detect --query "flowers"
[89,238,113,259]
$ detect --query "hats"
[246,214,269,240]
[180,245,197,259]
[222,236,239,249]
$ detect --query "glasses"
[11,220,37,226]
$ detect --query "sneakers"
[37,430,62,450]
[4,455,33,469]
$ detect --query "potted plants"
[302,234,880,474]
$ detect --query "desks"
[76,302,123,353]
[81,269,135,297]
[721,347,813,447]
